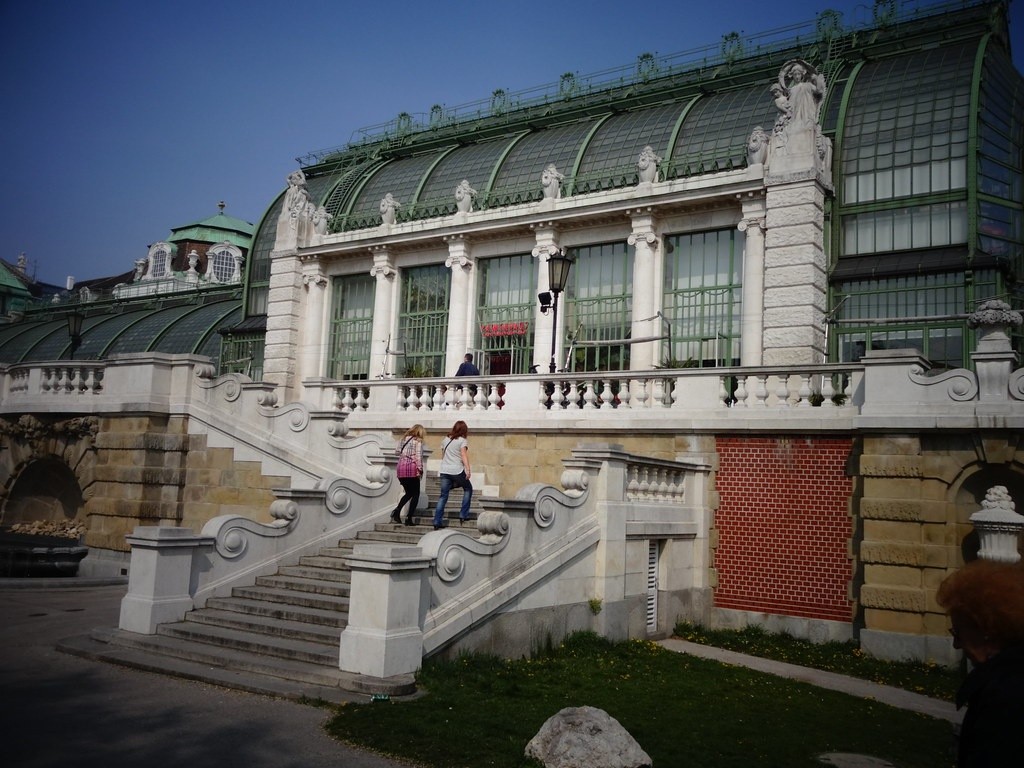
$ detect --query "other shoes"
[460,518,470,522]
[434,524,444,530]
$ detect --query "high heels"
[405,517,416,526]
[389,509,403,525]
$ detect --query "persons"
[433,421,473,530]
[280,169,332,230]
[770,65,825,155]
[455,354,480,393]
[390,425,426,526]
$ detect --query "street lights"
[546,249,572,408]
[67,307,85,390]
